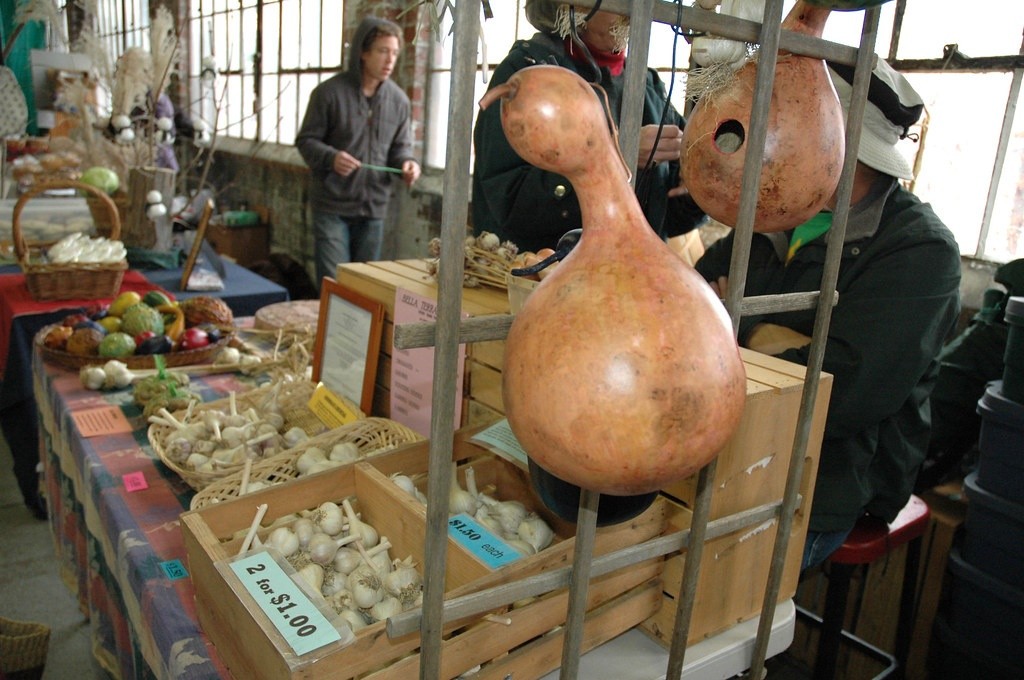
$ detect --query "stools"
[793,494,930,680]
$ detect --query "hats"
[827,55,926,181]
[525,0,567,40]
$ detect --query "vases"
[85,194,132,240]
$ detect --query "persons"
[473,0,706,261]
[130,92,181,176]
[690,52,963,570]
[296,16,422,301]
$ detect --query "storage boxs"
[177,415,668,680]
[337,259,833,650]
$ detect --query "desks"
[32,317,312,680]
[205,218,270,259]
[0,256,288,521]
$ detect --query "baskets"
[13,181,129,300]
[33,310,233,372]
[87,194,170,249]
[148,379,367,492]
[189,416,428,515]
[0,618,51,680]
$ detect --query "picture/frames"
[311,276,387,416]
